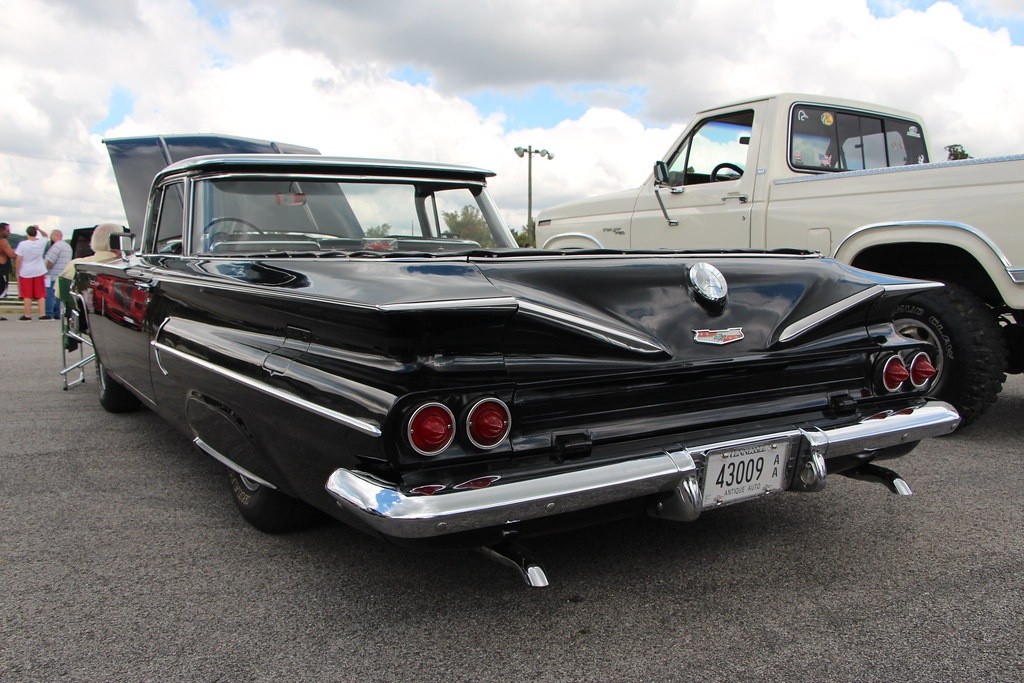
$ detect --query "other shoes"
[0,317,8,321]
[39,316,51,320]
[19,315,31,320]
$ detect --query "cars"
[64,135,962,586]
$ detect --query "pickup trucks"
[532,92,1023,433]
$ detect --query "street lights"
[514,145,554,247]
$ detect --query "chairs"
[59,275,96,393]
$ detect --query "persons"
[0,222,124,322]
